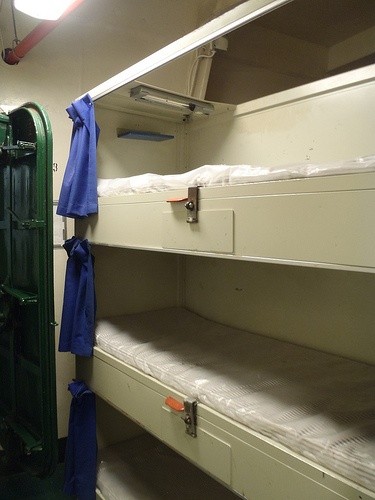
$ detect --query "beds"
[76,305,375,500]
[76,158,375,275]
